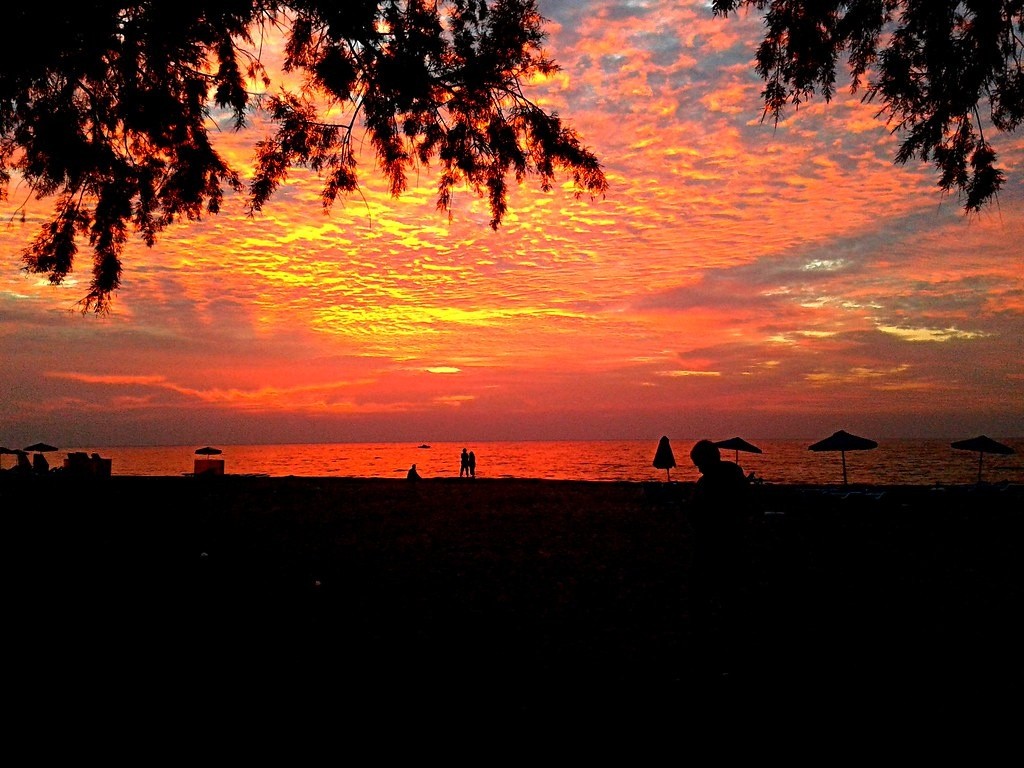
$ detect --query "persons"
[808,430,878,482]
[460,448,476,478]
[407,464,422,479]
[747,471,758,479]
[691,440,750,678]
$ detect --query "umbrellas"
[951,435,1014,481]
[0,443,59,465]
[653,436,676,481]
[715,437,762,465]
[195,447,221,460]
